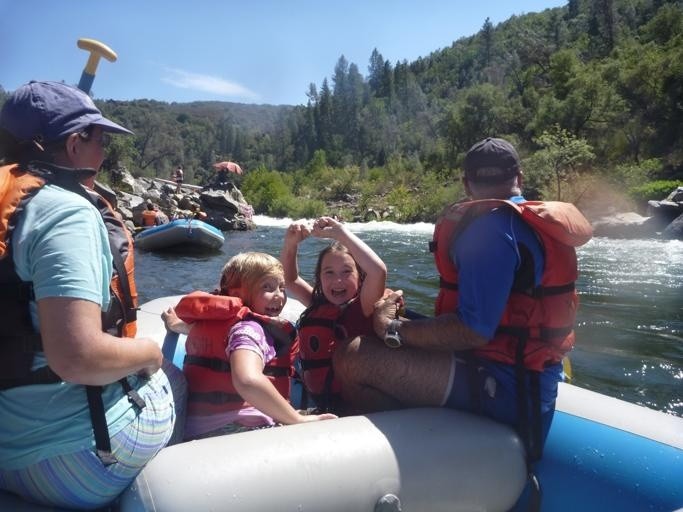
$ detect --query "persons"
[138,203,160,230]
[174,164,183,194]
[217,167,228,182]
[190,203,200,219]
[0,77,189,512]
[177,212,187,219]
[280,212,388,416]
[167,212,176,222]
[161,252,338,440]
[330,135,591,424]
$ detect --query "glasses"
[91,133,112,148]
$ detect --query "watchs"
[381,320,404,351]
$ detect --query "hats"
[464,138,521,182]
[1,79,133,144]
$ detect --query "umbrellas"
[211,160,242,175]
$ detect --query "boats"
[132,218,225,251]
[117,294,683,512]
